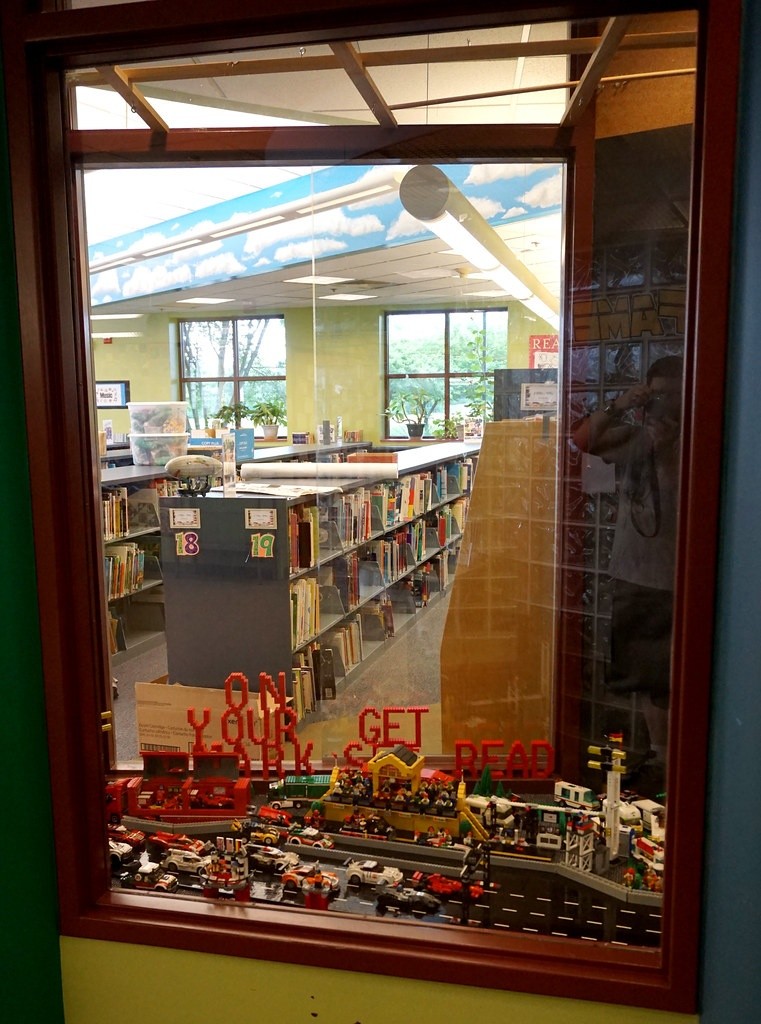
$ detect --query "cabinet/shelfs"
[97,434,484,743]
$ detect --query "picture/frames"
[95,380,130,410]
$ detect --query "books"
[112,433,129,443]
[289,456,480,723]
[98,431,106,456]
[278,428,366,463]
[102,461,116,469]
[102,478,244,651]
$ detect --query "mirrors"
[40,1,739,1016]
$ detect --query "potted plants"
[251,400,285,440]
[383,390,444,438]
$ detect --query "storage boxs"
[127,434,188,466]
[126,402,187,434]
[134,680,295,763]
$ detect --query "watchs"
[602,399,618,418]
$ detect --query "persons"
[211,851,220,872]
[265,788,275,802]
[333,771,457,845]
[277,814,285,825]
[622,858,663,893]
[219,856,226,870]
[313,870,322,891]
[524,806,535,839]
[313,810,320,828]
[464,834,472,845]
[572,356,682,783]
[222,868,232,886]
